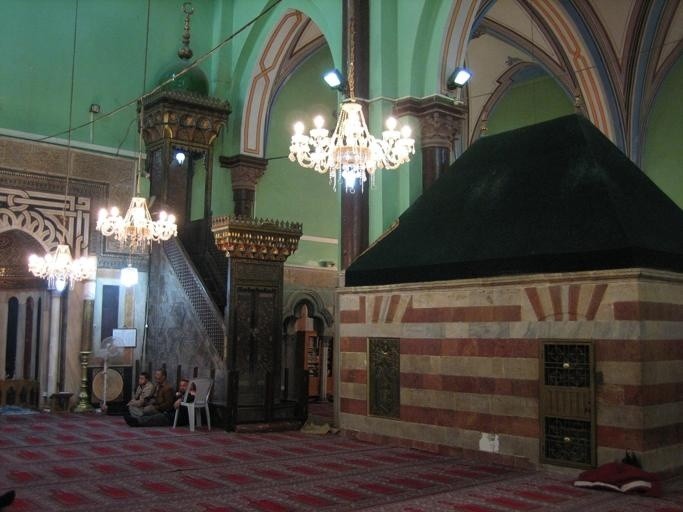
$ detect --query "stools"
[49,392,73,416]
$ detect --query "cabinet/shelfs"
[296,330,333,398]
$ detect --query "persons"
[100,372,154,416]
[125,380,195,426]
[139,369,175,416]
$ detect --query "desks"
[1,378,39,411]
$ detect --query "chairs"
[171,375,214,431]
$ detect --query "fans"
[94,336,125,413]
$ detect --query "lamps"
[321,69,345,91]
[449,66,475,88]
[95,1,181,251]
[287,0,419,193]
[28,1,100,291]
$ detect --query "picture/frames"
[112,327,137,348]
